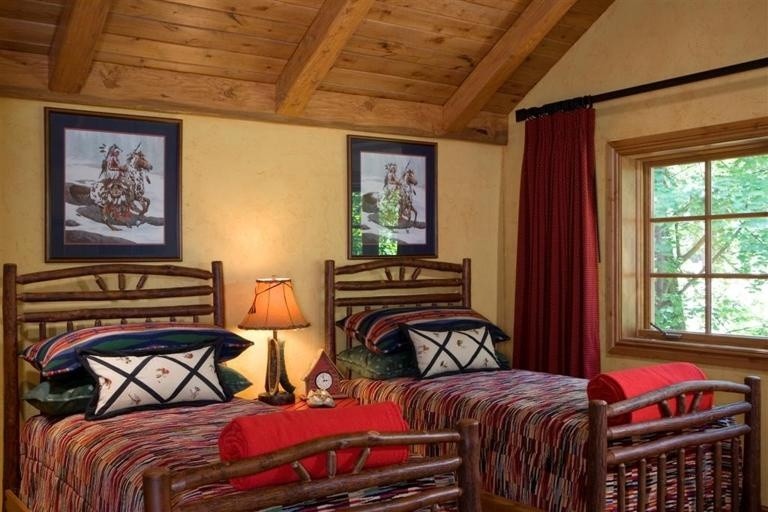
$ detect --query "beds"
[2,258,484,511]
[325,260,762,510]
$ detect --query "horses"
[64,152,152,231]
[361,169,417,228]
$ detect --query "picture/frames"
[42,105,183,265]
[346,134,439,261]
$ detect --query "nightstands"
[259,394,360,412]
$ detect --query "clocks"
[304,349,347,399]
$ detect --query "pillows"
[18,322,255,384]
[21,364,254,419]
[76,338,236,423]
[397,319,511,380]
[218,401,411,491]
[335,306,511,355]
[335,345,511,381]
[586,362,716,426]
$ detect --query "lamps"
[238,276,313,406]
